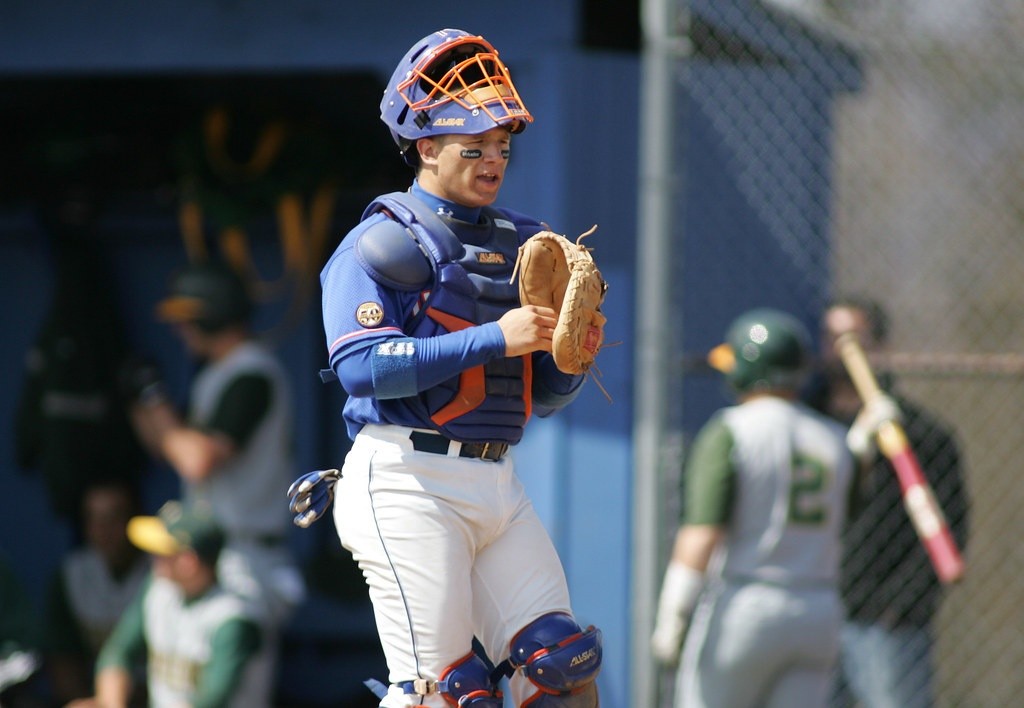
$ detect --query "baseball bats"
[835,334,964,587]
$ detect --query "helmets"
[707,307,813,391]
[380,28,527,168]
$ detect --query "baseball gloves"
[515,231,611,376]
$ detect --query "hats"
[159,269,245,324]
[126,500,224,567]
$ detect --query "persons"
[51,266,312,708]
[319,27,606,708]
[653,295,967,708]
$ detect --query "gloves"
[287,469,343,529]
[650,562,705,668]
[846,390,906,466]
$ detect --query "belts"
[409,431,509,462]
